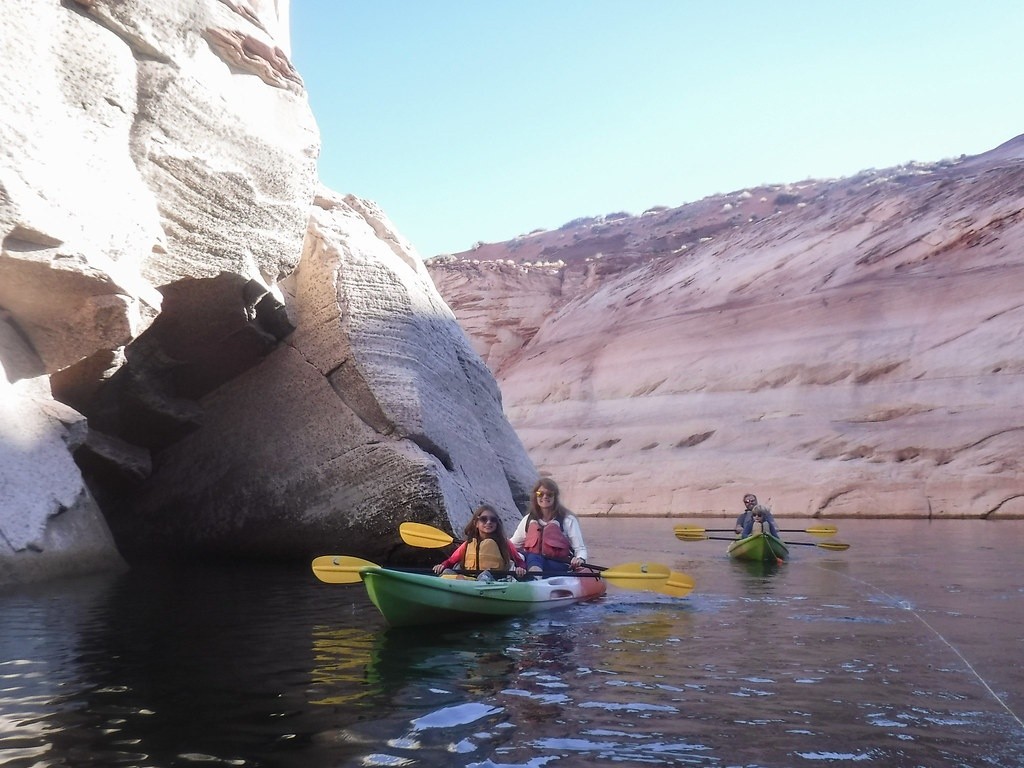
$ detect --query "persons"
[510,479,587,578]
[742,504,780,539]
[736,494,780,533]
[433,505,526,581]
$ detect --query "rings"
[577,561,578,562]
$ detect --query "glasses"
[744,499,756,503]
[477,516,499,522]
[752,513,763,517]
[536,491,554,498]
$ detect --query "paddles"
[398,520,696,599]
[673,523,851,552]
[308,553,672,592]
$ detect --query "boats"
[727,530,792,564]
[359,566,610,628]
